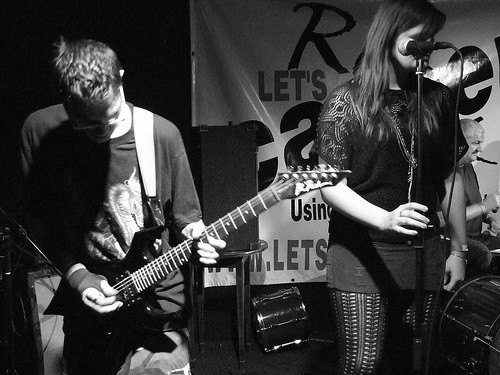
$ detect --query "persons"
[314,0,465,375]
[433,118,500,274]
[13,36,227,375]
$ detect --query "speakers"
[182,123,261,252]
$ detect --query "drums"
[252,287,311,354]
[432,275,500,375]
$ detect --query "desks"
[186,240,268,369]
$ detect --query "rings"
[398,210,402,217]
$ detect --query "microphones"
[398,37,452,56]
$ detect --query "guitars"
[43,164,353,375]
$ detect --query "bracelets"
[448,242,472,252]
[476,201,489,215]
[446,252,470,265]
